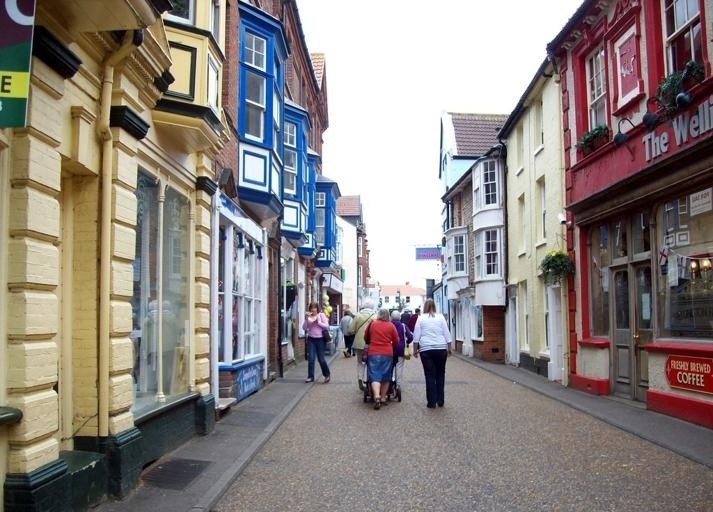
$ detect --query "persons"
[339,298,454,409]
[302,301,331,383]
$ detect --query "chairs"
[325,375,330,382]
[306,377,313,382]
[358,378,365,391]
[343,349,351,357]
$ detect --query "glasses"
[676,72,713,110]
[643,97,674,133]
[614,118,636,146]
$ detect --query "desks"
[356,224,363,312]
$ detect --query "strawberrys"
[364,354,401,402]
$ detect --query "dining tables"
[683,60,704,83]
[574,126,608,157]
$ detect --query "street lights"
[322,330,333,345]
[404,347,410,360]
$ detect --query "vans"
[374,398,387,409]
